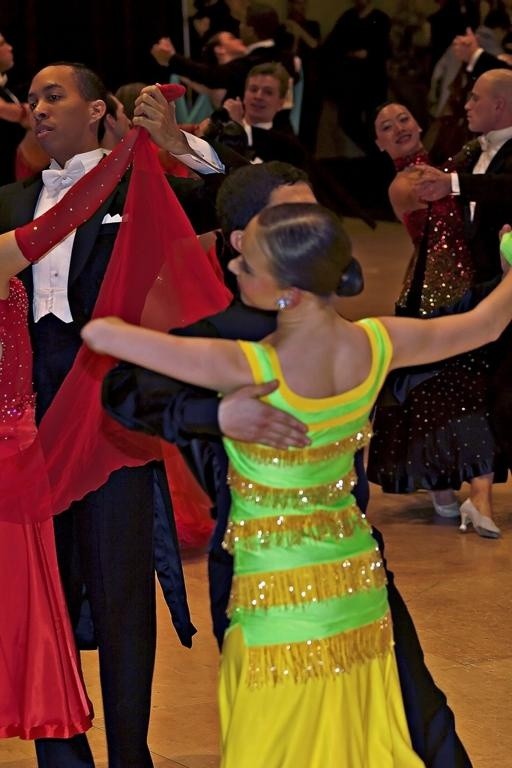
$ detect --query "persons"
[0,1,512,768]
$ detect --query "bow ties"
[42,159,85,194]
[477,136,488,152]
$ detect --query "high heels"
[460,497,501,538]
[428,487,460,517]
